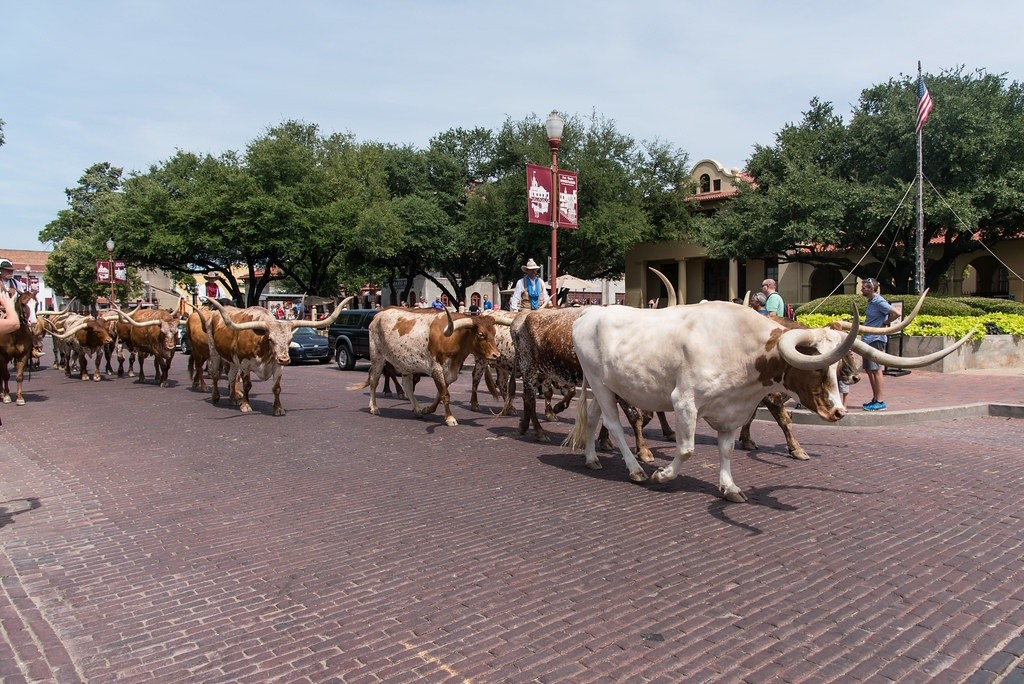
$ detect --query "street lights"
[24,263,31,292]
[106,238,116,310]
[544,114,570,310]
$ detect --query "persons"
[483,295,492,310]
[751,279,784,317]
[573,299,579,306]
[199,272,226,302]
[375,304,380,310]
[432,297,443,309]
[510,258,554,312]
[830,322,854,413]
[0,258,45,357]
[862,278,902,411]
[401,296,428,308]
[271,299,306,320]
[469,301,479,316]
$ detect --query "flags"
[916,73,934,134]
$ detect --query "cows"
[0,289,39,405]
[201,296,354,416]
[468,267,677,463]
[183,301,230,393]
[560,301,979,502]
[97,300,141,377]
[26,296,78,372]
[43,301,143,382]
[106,295,183,377]
[346,293,556,426]
[109,309,188,388]
[656,288,928,459]
[37,312,81,369]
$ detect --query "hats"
[521,259,541,272]
[0,261,15,270]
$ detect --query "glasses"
[867,278,874,290]
[762,284,770,288]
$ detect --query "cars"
[289,327,335,364]
[175,319,191,355]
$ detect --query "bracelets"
[883,323,888,325]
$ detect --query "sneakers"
[863,401,887,411]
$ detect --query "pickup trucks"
[328,309,464,372]
